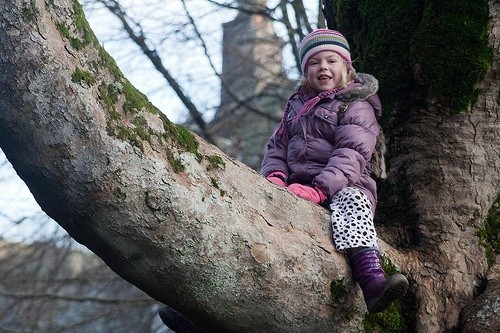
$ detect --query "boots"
[158,305,200,333]
[350,248,409,313]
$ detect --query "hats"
[299,29,352,76]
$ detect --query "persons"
[159,28,408,333]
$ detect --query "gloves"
[267,171,288,187]
[287,183,328,205]
